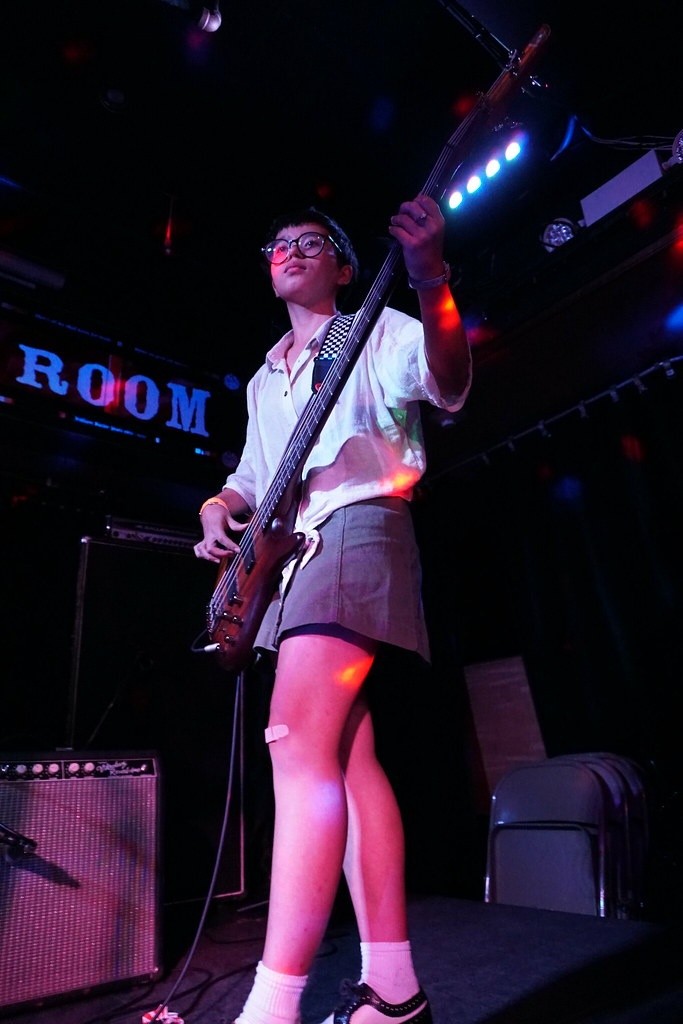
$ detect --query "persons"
[194,193,473,1024]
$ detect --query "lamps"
[398,242,467,296]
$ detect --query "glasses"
[260,232,344,265]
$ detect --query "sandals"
[328,980,432,1024]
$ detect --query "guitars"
[204,24,564,674]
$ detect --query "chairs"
[480,750,646,918]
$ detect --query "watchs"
[408,260,451,290]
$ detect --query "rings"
[416,212,428,226]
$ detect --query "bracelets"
[200,497,229,514]
